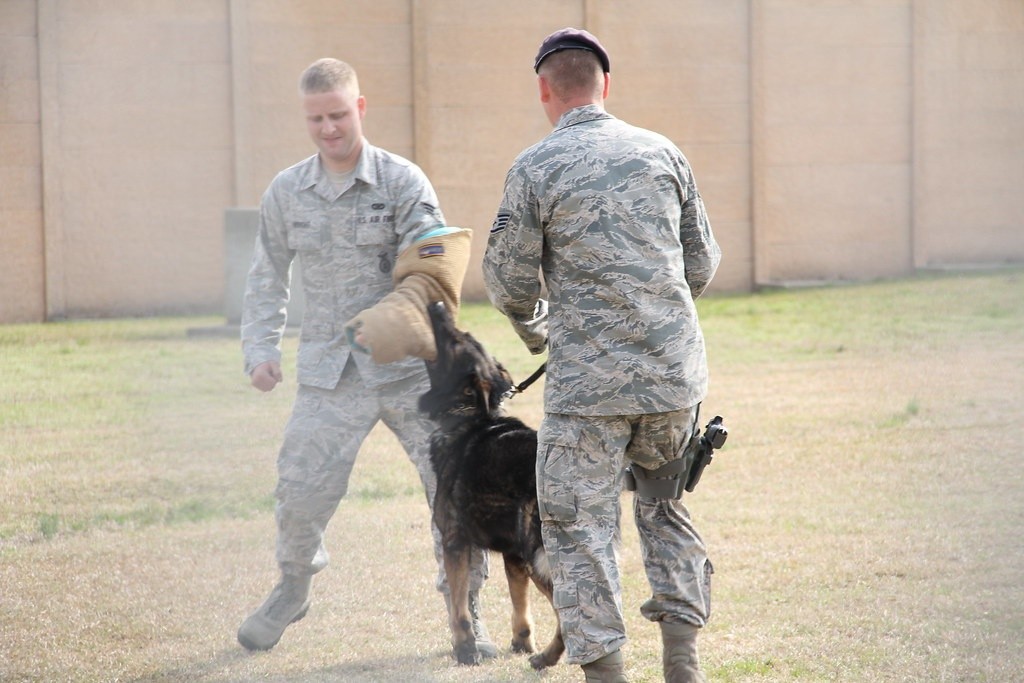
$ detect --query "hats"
[534,28,610,74]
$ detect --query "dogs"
[418,299,566,671]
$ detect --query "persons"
[240,57,499,658]
[483,28,722,683]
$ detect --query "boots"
[238,576,315,650]
[660,621,705,683]
[581,651,628,683]
[445,591,495,658]
